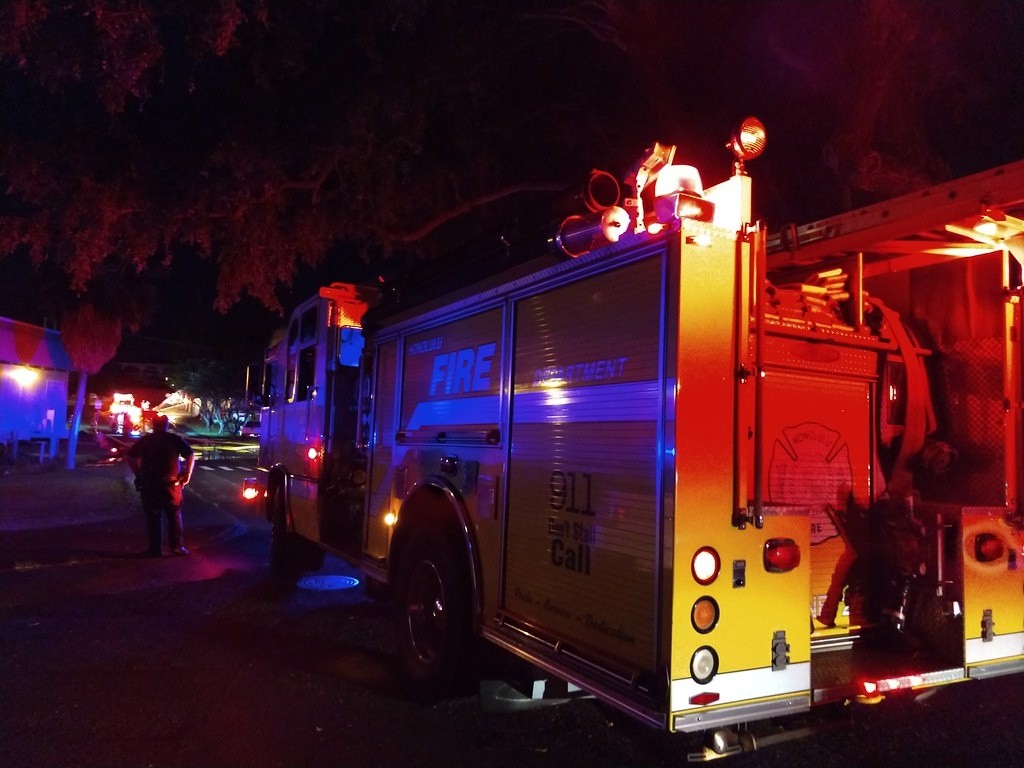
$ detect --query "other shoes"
[174,546,189,555]
[141,548,161,557]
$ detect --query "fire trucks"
[242,115,1024,762]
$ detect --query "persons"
[68,413,74,428]
[126,414,195,557]
[86,392,101,435]
